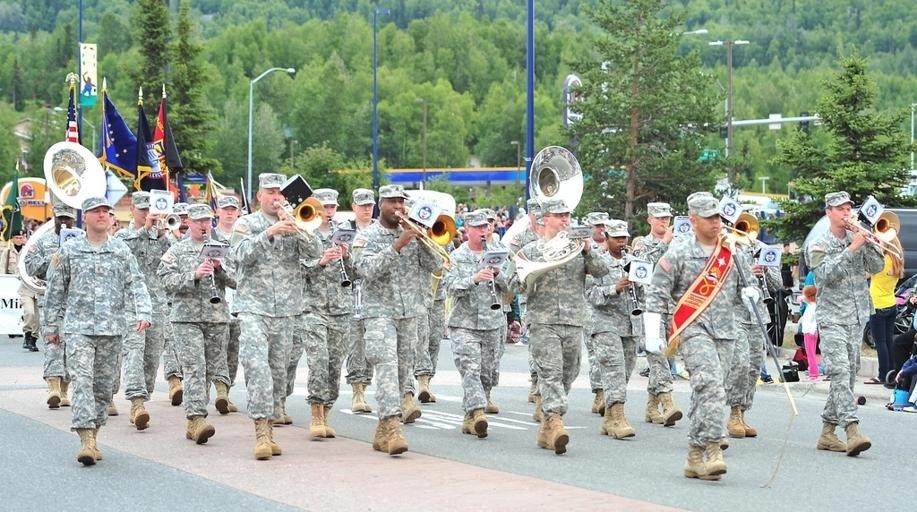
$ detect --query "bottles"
[587,212,609,225]
[824,190,855,208]
[312,185,409,205]
[475,208,496,220]
[52,191,149,220]
[172,197,239,219]
[541,199,569,213]
[11,229,24,236]
[258,172,287,189]
[646,192,721,218]
[464,214,490,227]
[526,198,543,217]
[604,220,630,238]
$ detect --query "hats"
[894,182,916,199]
[787,290,803,323]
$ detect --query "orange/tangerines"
[864,273,917,386]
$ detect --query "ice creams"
[152,214,183,232]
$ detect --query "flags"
[151,98,182,183]
[3,172,21,241]
[132,103,165,193]
[60,73,82,146]
[95,89,138,179]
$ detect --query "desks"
[412,98,426,185]
[757,176,770,194]
[678,28,711,40]
[371,7,395,191]
[708,38,750,188]
[247,66,298,203]
[508,140,520,184]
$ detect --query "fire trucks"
[482,235,501,309]
[750,245,773,304]
[201,228,222,304]
[325,216,352,287]
[619,245,641,315]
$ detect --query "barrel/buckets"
[864,377,883,384]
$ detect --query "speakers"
[45,379,60,408]
[526,382,568,454]
[460,389,498,438]
[92,425,101,460]
[309,383,371,438]
[846,422,871,456]
[167,377,214,445]
[685,403,756,481]
[75,428,95,465]
[372,374,436,454]
[227,388,237,412]
[253,398,292,459]
[816,421,846,453]
[59,381,70,406]
[887,390,910,409]
[106,396,149,430]
[592,389,682,439]
[23,331,30,348]
[213,381,229,414]
[27,337,37,352]
[885,389,899,407]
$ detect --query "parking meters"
[842,210,903,261]
[274,197,327,244]
[722,213,759,247]
[394,211,456,265]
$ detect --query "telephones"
[699,150,722,161]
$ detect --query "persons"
[25,202,87,408]
[647,195,763,479]
[584,219,650,435]
[797,227,914,414]
[451,200,798,410]
[157,204,240,445]
[42,197,152,465]
[807,191,886,455]
[516,199,608,453]
[230,173,324,458]
[3,217,51,351]
[352,184,442,455]
[109,189,375,423]
[299,189,358,440]
[446,211,517,438]
[724,233,783,438]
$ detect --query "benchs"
[95,89,138,179]
[132,103,165,193]
[60,73,82,146]
[151,98,182,183]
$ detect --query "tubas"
[531,144,583,214]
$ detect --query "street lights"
[779,360,800,382]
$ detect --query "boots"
[794,208,917,351]
